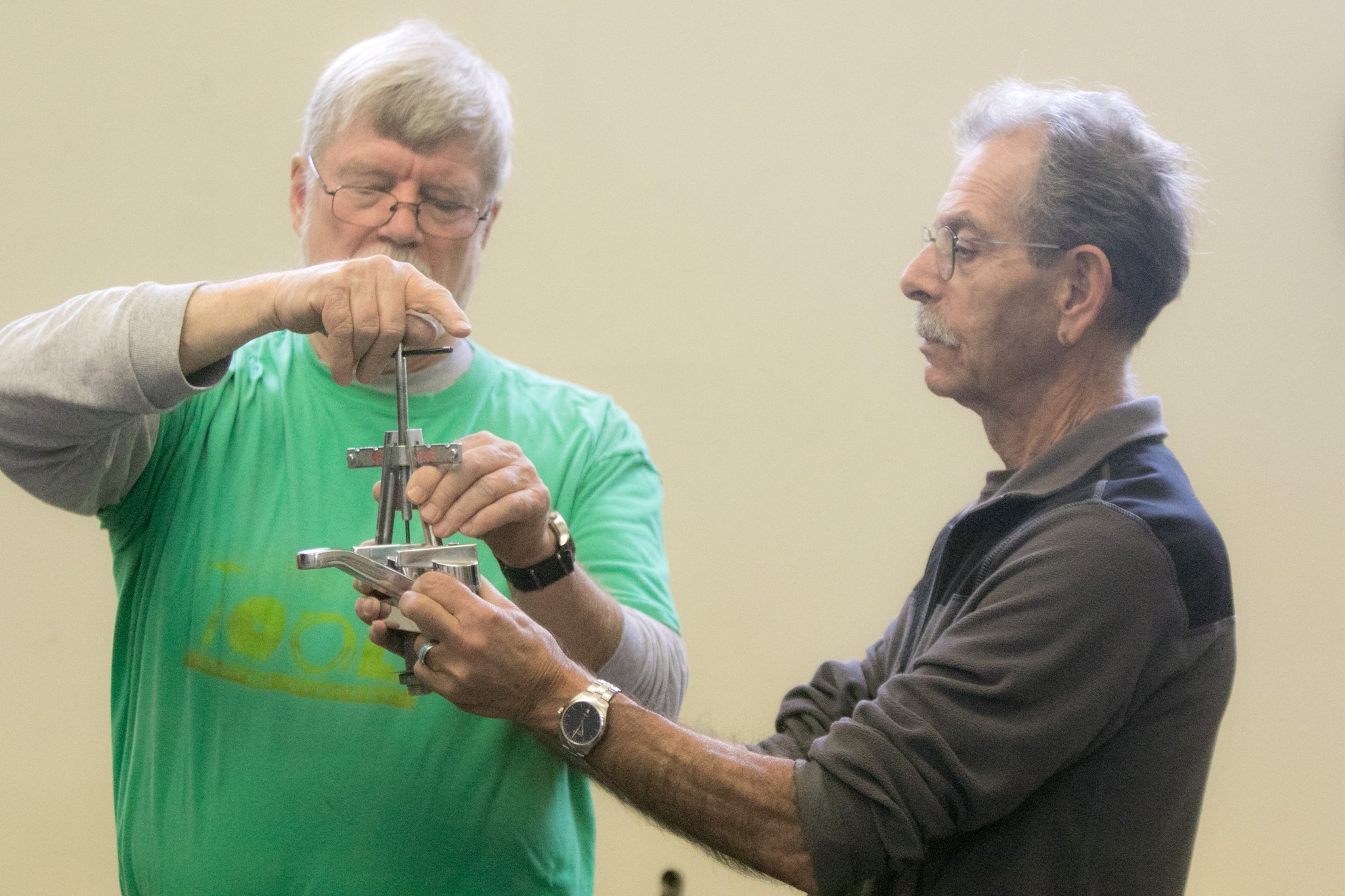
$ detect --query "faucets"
[293,541,479,700]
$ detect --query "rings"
[418,641,436,668]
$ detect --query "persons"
[0,27,688,896]
[350,87,1234,896]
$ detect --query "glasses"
[307,153,492,241]
[922,225,1065,281]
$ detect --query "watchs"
[496,511,576,592]
[557,677,623,768]
